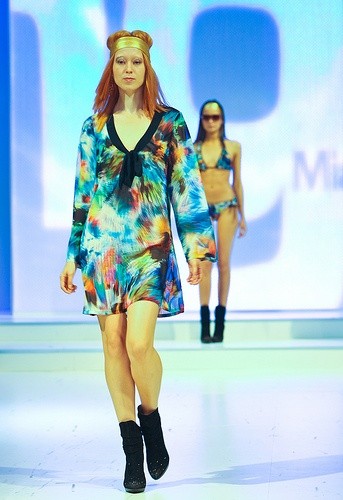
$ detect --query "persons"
[61,29,216,493]
[191,99,246,343]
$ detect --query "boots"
[138,404,169,480]
[119,419,146,493]
[200,304,212,343]
[212,304,226,342]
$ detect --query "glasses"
[201,114,221,121]
